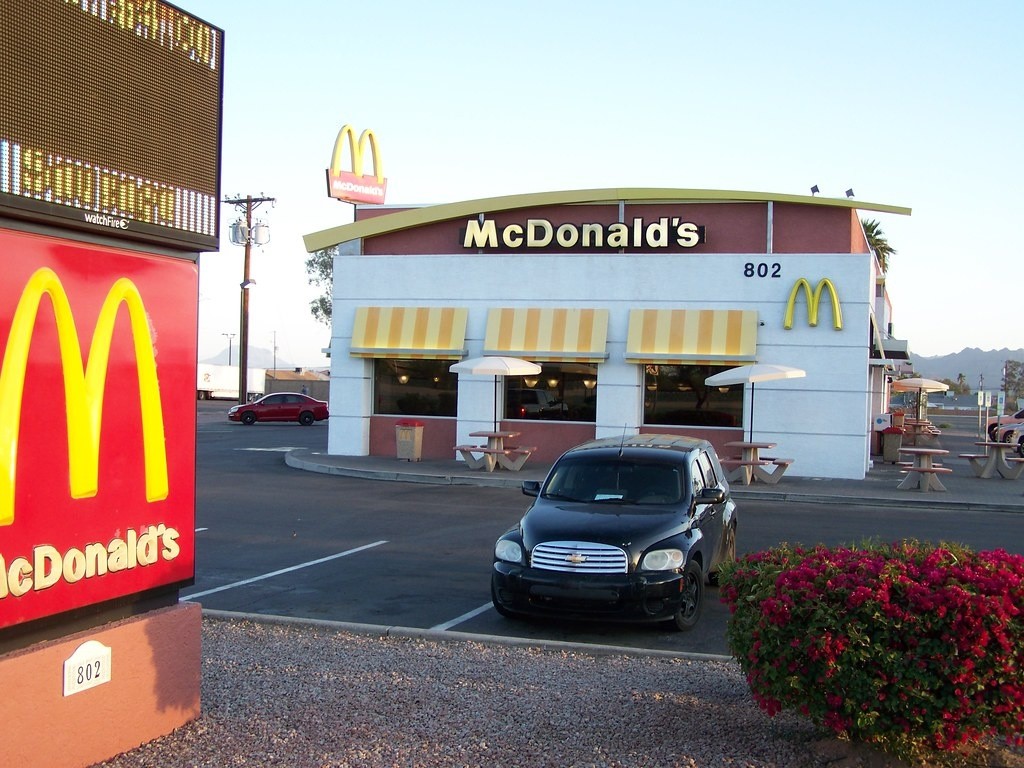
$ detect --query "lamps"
[582,379,596,388]
[646,385,657,392]
[547,379,560,387]
[523,376,539,388]
[397,375,410,384]
[719,387,730,393]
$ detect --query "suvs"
[491,435,739,632]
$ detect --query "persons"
[301,385,309,396]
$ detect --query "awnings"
[626,308,758,368]
[349,306,466,361]
[485,308,610,363]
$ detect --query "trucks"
[197,363,268,401]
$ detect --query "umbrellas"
[892,377,949,423]
[449,355,542,434]
[704,362,806,443]
[539,362,598,411]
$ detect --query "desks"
[974,442,1021,478]
[905,422,930,446]
[469,431,520,469]
[897,447,949,492]
[724,441,776,481]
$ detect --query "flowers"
[714,535,1024,758]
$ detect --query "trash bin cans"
[891,411,905,427]
[881,424,904,462]
[393,418,425,463]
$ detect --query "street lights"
[221,332,236,366]
[221,193,277,406]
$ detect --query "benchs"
[719,456,795,486]
[958,454,1024,480]
[452,445,537,471]
[895,417,952,491]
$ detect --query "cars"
[990,421,1024,443]
[985,409,1024,442]
[1011,422,1024,457]
[227,392,329,425]
